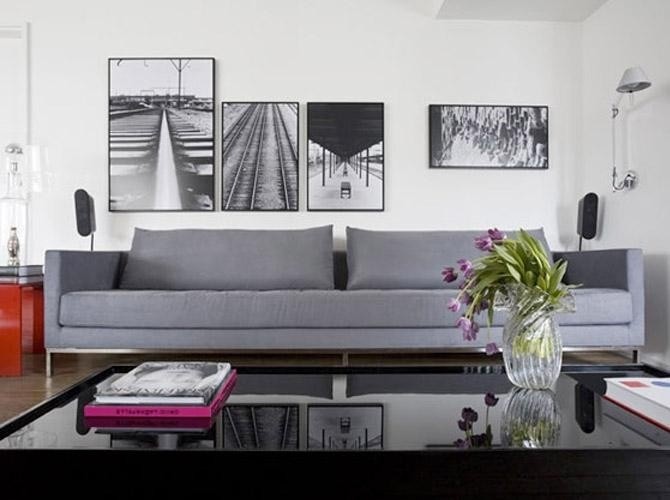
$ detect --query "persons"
[441,106,547,168]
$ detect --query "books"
[604,376,670,433]
[83,366,238,417]
[83,417,214,430]
[603,400,670,447]
[93,360,230,406]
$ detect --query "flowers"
[454,390,499,449]
[441,226,585,360]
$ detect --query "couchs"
[43,249,646,380]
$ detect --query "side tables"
[1,284,43,376]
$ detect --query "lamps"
[0,145,28,266]
[610,67,652,194]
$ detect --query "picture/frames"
[428,104,549,170]
[221,101,385,212]
[108,401,386,451]
[108,57,215,213]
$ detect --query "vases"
[503,306,562,389]
[500,390,564,449]
